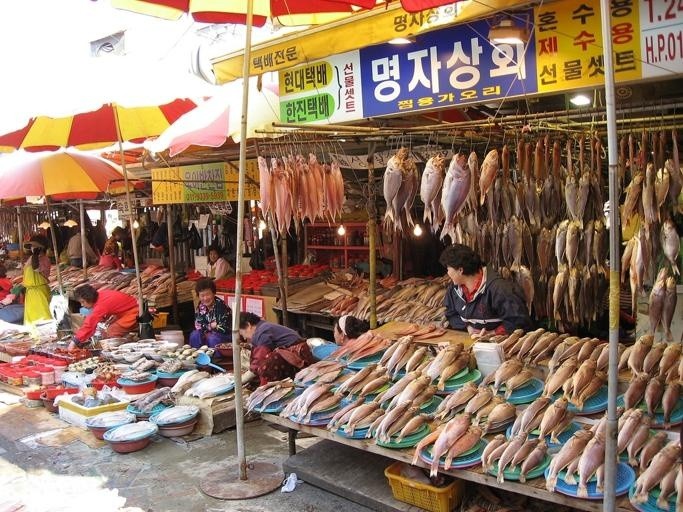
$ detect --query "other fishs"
[245,126,682,341]
[245,126,683,511]
[244,320,682,512]
[49,263,187,300]
[129,368,211,413]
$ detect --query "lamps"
[37,206,50,230]
[486,12,530,45]
[132,204,139,230]
[259,216,267,232]
[335,216,346,236]
[64,205,78,229]
[413,214,422,238]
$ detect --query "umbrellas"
[0,148,145,294]
[0,48,215,319]
[152,79,302,328]
[97,0,473,477]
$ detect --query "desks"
[256,318,682,512]
[139,287,190,327]
[276,273,383,340]
[191,269,321,340]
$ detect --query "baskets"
[384,460,466,512]
[152,312,169,329]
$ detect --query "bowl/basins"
[23,387,80,414]
[214,342,233,357]
[85,338,199,454]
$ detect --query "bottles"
[310,228,335,245]
[350,230,363,246]
[0,354,66,386]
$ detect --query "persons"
[438,244,532,337]
[332,316,363,347]
[19,211,235,357]
[239,311,313,387]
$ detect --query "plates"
[247,339,682,512]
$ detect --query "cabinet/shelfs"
[299,210,389,273]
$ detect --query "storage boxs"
[54,393,131,431]
